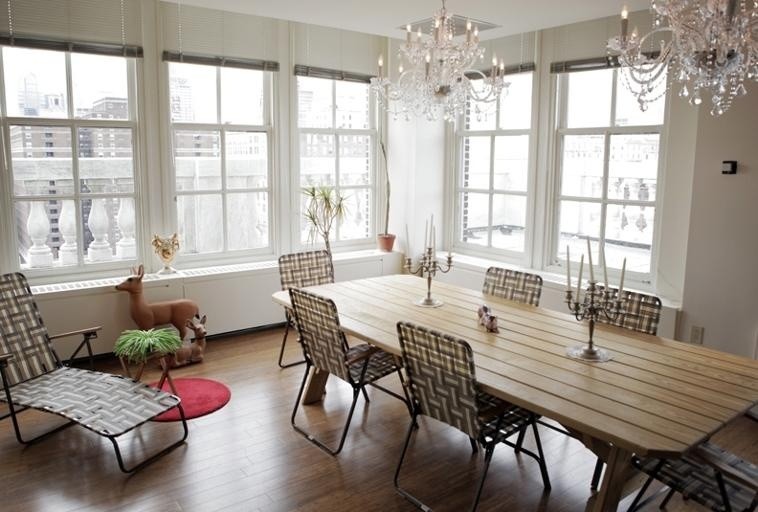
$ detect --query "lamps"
[604,0,758,119]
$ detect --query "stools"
[119,352,180,395]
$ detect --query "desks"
[272,274,758,511]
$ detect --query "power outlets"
[688,325,704,345]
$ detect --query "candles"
[566,240,626,305]
[402,214,454,260]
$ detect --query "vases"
[377,232,395,252]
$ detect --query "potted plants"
[113,327,182,365]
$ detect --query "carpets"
[145,377,231,422]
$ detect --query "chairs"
[582,285,662,487]
[291,287,419,455]
[368,0,514,125]
[0,272,188,474]
[482,266,543,308]
[278,249,334,369]
[393,320,551,512]
[626,441,757,512]
